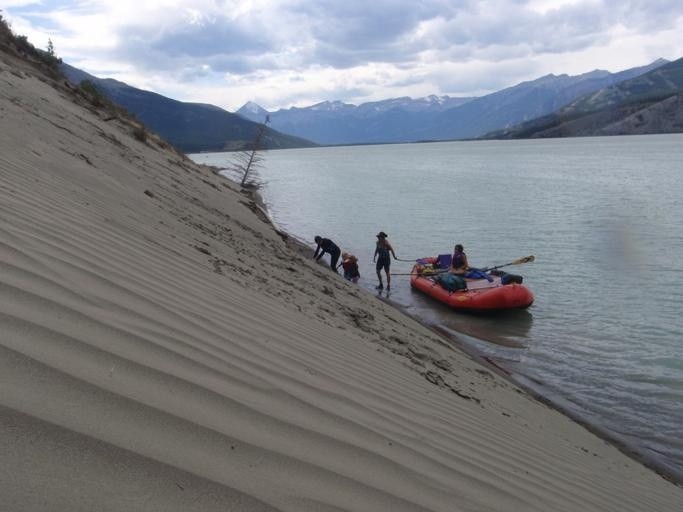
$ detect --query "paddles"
[483,256,534,273]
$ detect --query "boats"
[416,290,534,338]
[409,255,536,311]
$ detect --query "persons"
[372,231,396,291]
[343,255,360,284]
[447,244,468,275]
[312,234,341,276]
[335,250,351,284]
